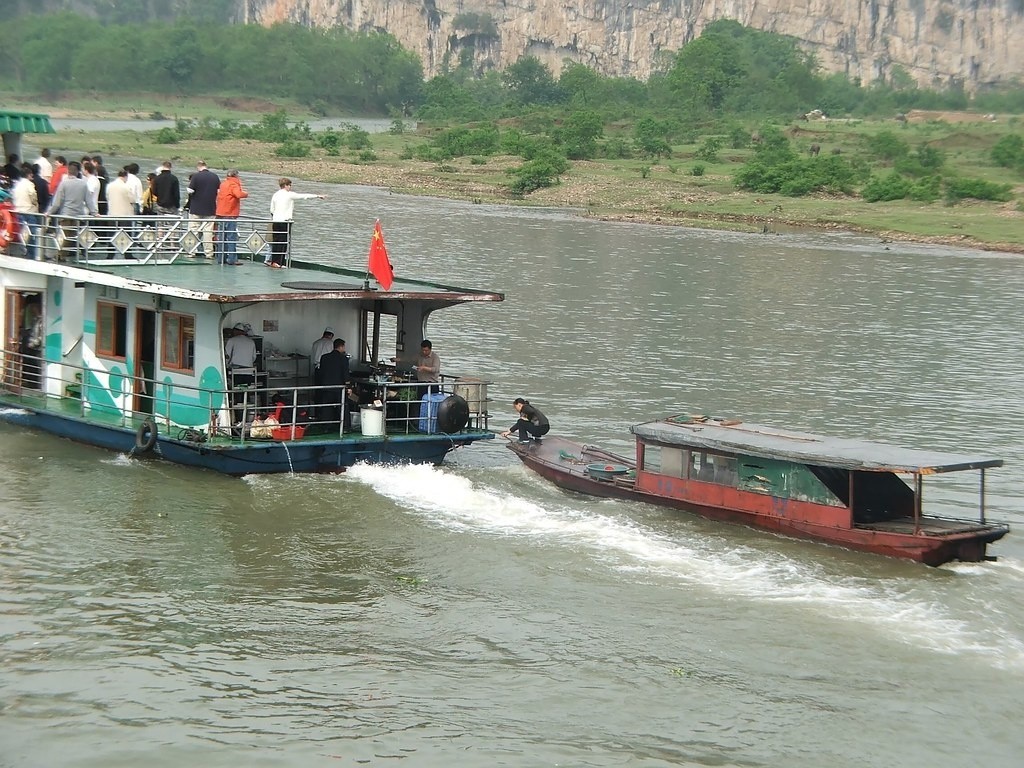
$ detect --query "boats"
[0,107,511,483]
[506,414,1012,569]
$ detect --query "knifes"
[345,389,359,402]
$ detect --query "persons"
[271,393,310,429]
[17,291,42,388]
[224,321,257,421]
[318,337,352,434]
[263,222,274,266]
[270,178,330,271]
[216,168,249,266]
[500,397,550,443]
[311,327,335,418]
[392,339,441,431]
[0,148,218,261]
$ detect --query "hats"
[325,327,336,334]
[234,322,245,330]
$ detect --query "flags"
[368,218,394,291]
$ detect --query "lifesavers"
[0,209,14,246]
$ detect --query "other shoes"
[517,440,529,445]
[264,260,272,267]
[272,262,287,268]
[228,261,244,264]
[529,436,541,440]
[219,261,227,264]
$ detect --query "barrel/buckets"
[453,380,487,413]
[360,408,384,436]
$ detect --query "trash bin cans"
[358,402,388,436]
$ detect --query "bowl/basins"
[437,395,469,434]
[586,464,630,482]
[271,426,306,440]
